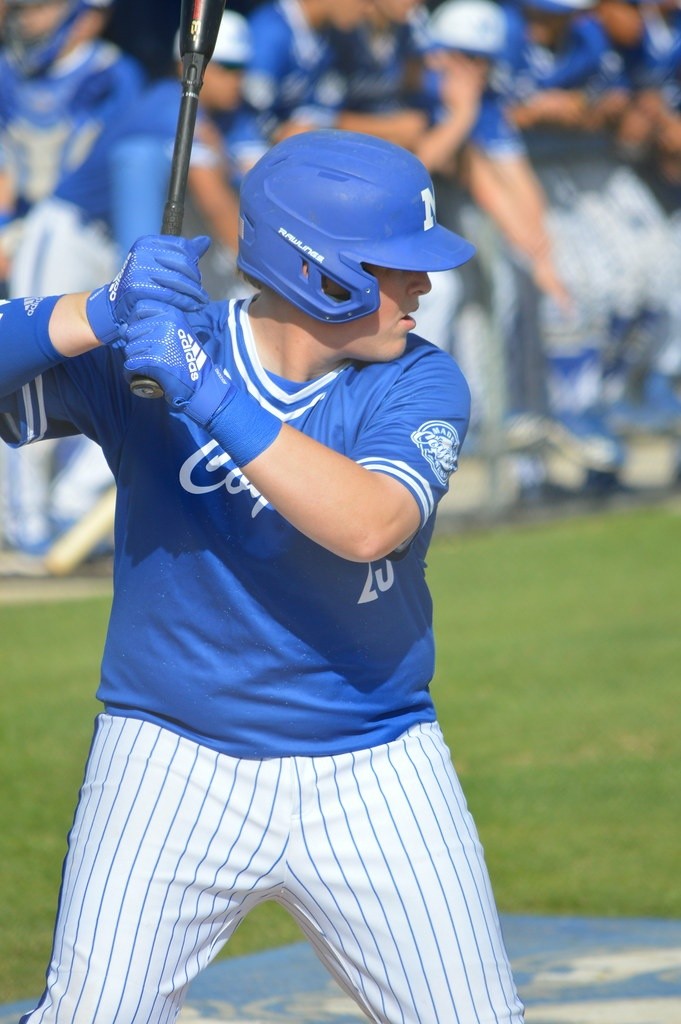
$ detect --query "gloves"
[86,235,213,349]
[121,298,239,429]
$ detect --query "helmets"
[230,126,474,326]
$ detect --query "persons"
[0,127,526,1023]
[1,2,679,572]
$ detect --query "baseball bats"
[129,0,226,401]
